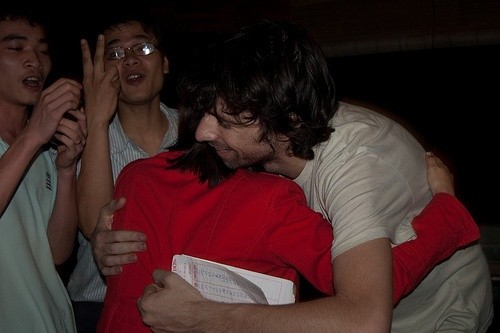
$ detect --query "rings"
[76,141,82,145]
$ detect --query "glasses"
[105,42,159,59]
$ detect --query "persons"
[90,19,494,333]
[96,70,481,333]
[66,14,179,333]
[0,10,87,333]
